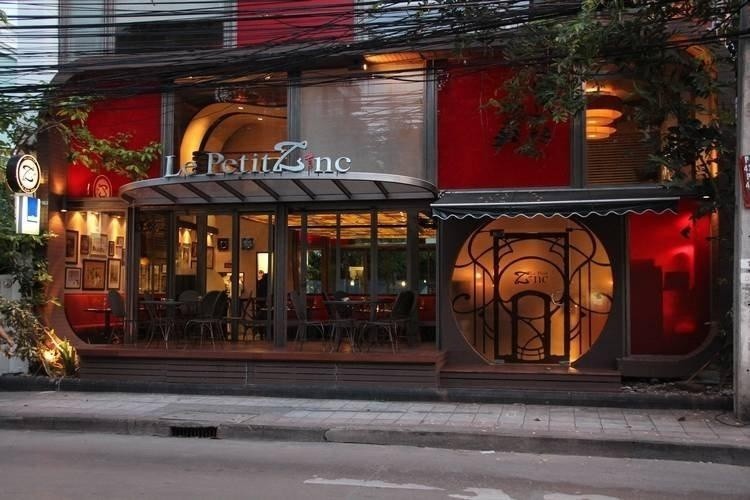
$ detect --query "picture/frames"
[65,267,81,290]
[207,246,215,269]
[82,259,106,290]
[116,236,124,247]
[218,237,229,250]
[240,237,255,250]
[80,235,89,254]
[107,258,121,290]
[108,240,115,257]
[65,230,79,266]
[90,232,108,256]
[191,241,198,257]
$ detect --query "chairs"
[86,290,442,355]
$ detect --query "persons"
[228,276,243,300]
[259,271,267,291]
